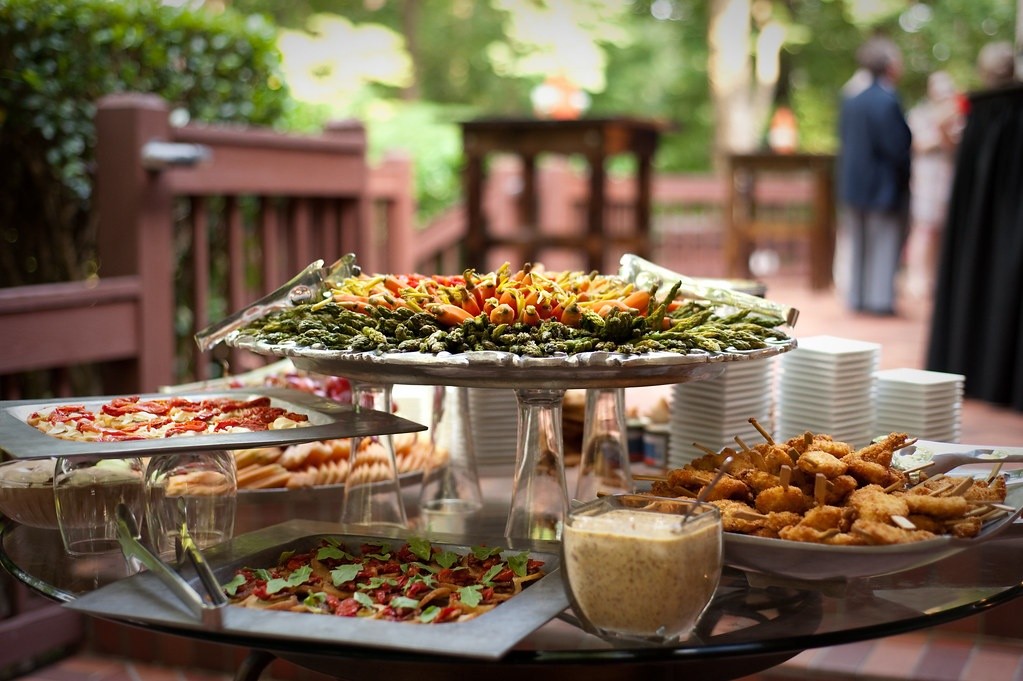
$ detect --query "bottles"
[418,385,491,533]
[339,380,409,530]
[574,388,635,507]
[504,387,570,541]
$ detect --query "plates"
[61,520,575,658]
[724,469,1023,580]
[777,335,880,450]
[451,386,517,479]
[871,368,966,444]
[668,357,775,469]
[1,389,428,458]
[224,331,798,387]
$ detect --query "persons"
[922,41,1023,412]
[907,73,962,304]
[835,43,912,315]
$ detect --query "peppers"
[312,263,686,330]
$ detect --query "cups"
[564,494,720,645]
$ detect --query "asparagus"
[234,280,790,356]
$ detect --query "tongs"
[194,253,361,353]
[621,253,799,328]
[104,497,228,626]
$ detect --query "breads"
[156,434,449,495]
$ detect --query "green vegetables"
[219,536,531,623]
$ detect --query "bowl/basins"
[1,458,149,529]
[235,466,447,535]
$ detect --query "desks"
[455,115,676,275]
[723,153,840,289]
[0,448,1023,681]
